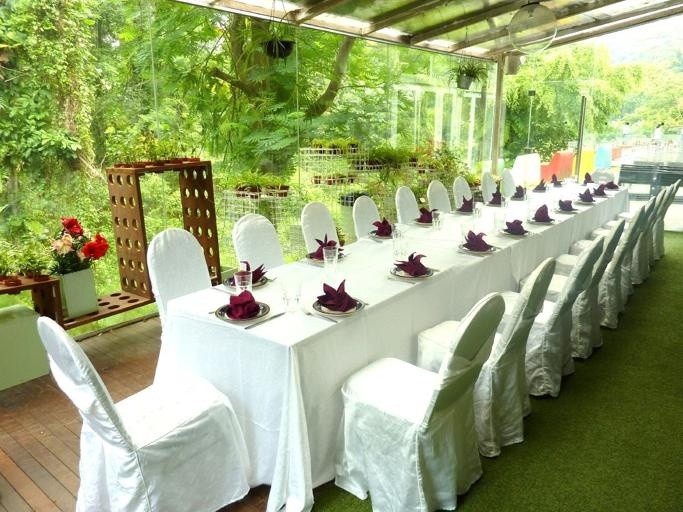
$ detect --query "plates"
[367,232,398,240]
[455,209,477,215]
[498,229,530,237]
[231,276,267,288]
[575,197,596,204]
[457,242,496,254]
[215,301,270,322]
[307,256,345,265]
[312,296,366,316]
[415,221,437,227]
[592,193,607,198]
[527,216,556,225]
[390,263,434,278]
[605,187,621,191]
[556,207,580,213]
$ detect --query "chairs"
[300,203,341,256]
[618,179,682,256]
[496,237,604,395]
[518,221,626,355]
[395,186,420,223]
[36,317,249,511]
[454,176,472,208]
[554,206,646,330]
[418,257,557,457]
[233,213,282,271]
[483,172,497,202]
[502,170,515,197]
[606,186,675,262]
[572,194,656,294]
[334,292,504,511]
[147,229,212,387]
[591,190,666,282]
[352,196,381,240]
[427,180,452,213]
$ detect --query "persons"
[653,123,665,141]
[622,122,630,137]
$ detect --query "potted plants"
[444,57,483,91]
[235,17,295,69]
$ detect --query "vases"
[56,269,99,316]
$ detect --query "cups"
[281,280,301,311]
[234,271,253,300]
[431,210,444,229]
[391,228,408,260]
[322,247,338,270]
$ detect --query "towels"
[593,184,605,196]
[462,230,490,252]
[577,188,593,202]
[510,185,526,201]
[312,232,344,260]
[487,185,502,205]
[394,252,428,277]
[606,182,618,189]
[559,199,573,211]
[456,195,473,212]
[223,290,261,321]
[371,217,392,237]
[228,260,268,286]
[584,172,594,184]
[316,279,357,312]
[533,178,546,192]
[550,174,562,187]
[414,207,438,223]
[503,220,527,236]
[532,205,554,222]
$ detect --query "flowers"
[50,218,109,275]
